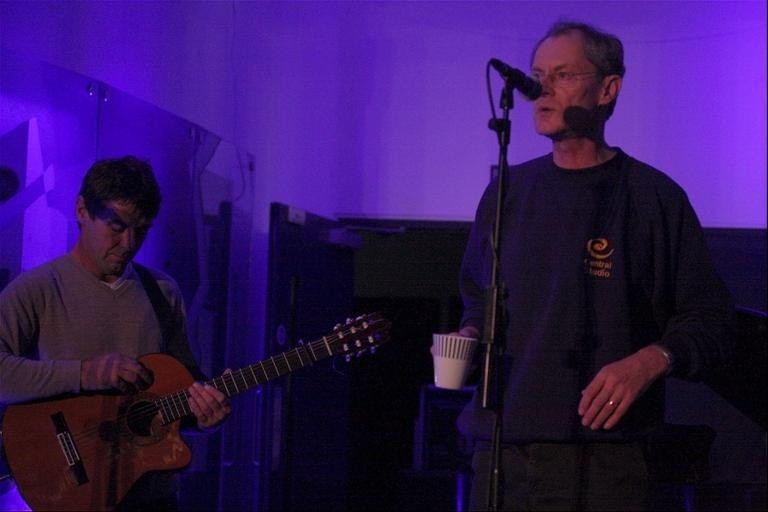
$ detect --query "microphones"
[490,58,542,101]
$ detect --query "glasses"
[525,70,601,87]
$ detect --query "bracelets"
[654,343,675,372]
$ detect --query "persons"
[0,157,231,512]
[431,21,738,509]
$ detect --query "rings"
[607,399,617,408]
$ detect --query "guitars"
[0,310,393,512]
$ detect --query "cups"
[433,332,479,391]
